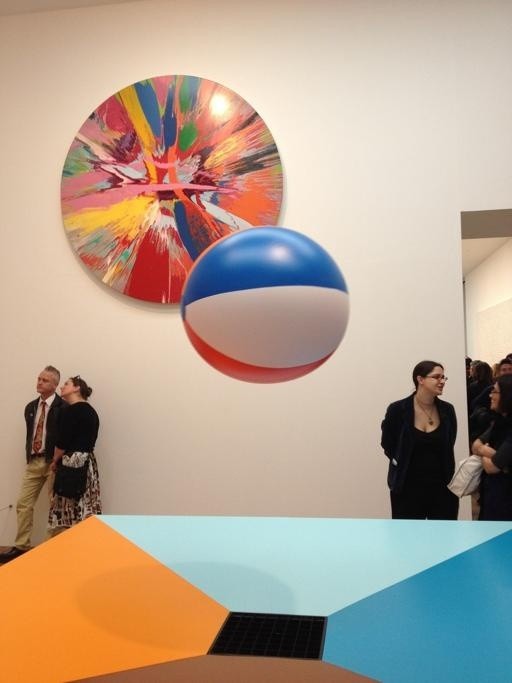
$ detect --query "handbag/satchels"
[54,465,87,497]
[447,454,483,498]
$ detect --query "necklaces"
[414,396,434,426]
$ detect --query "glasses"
[424,375,448,381]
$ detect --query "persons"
[381,361,459,519]
[466,354,512,521]
[47,375,103,538]
[0,365,63,563]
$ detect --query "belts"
[31,453,46,458]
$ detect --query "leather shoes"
[0,547,26,562]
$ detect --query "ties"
[33,403,46,454]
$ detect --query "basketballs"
[183,225,347,383]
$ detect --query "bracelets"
[51,457,59,467]
